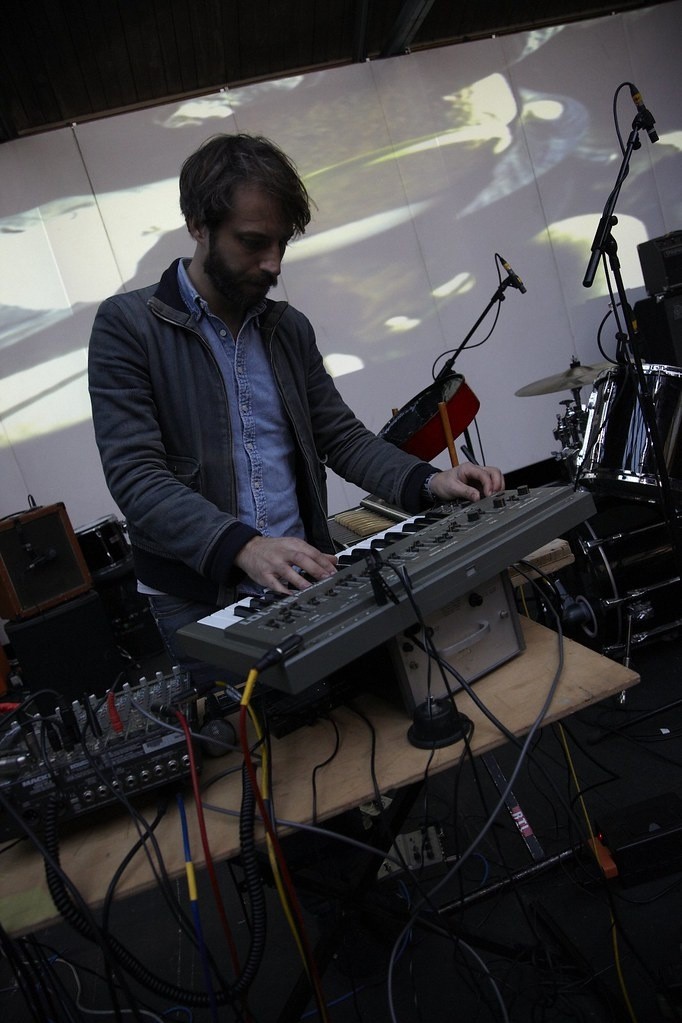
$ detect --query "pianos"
[323,499,413,545]
[177,484,598,695]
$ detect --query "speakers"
[4,592,126,719]
[0,502,90,620]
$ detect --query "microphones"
[501,257,526,293]
[629,83,658,143]
[369,560,469,747]
[198,696,236,755]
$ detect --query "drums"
[563,498,682,647]
[74,512,132,575]
[575,362,682,504]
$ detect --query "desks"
[1,609,645,1022]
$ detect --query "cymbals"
[513,353,646,395]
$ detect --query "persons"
[89,134,506,694]
